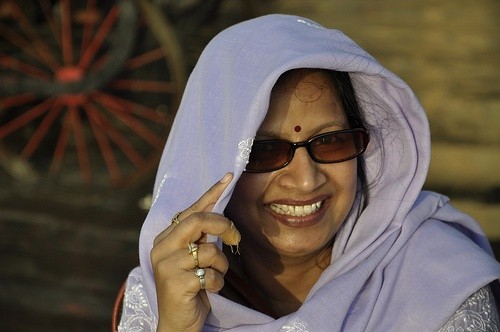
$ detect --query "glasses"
[242,128,371,175]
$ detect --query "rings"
[172,212,181,225]
[195,268,206,289]
[186,242,198,267]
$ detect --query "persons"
[117,12,500,332]
